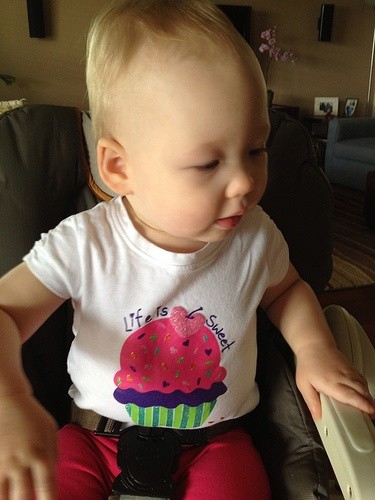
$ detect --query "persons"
[1,0,375,500]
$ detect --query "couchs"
[324,115,375,194]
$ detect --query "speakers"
[26,0,45,38]
[318,4,335,42]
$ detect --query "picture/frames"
[314,97,339,116]
[343,98,359,118]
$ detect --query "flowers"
[259,25,299,85]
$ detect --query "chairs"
[0,104,334,500]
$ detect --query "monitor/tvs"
[216,4,252,43]
